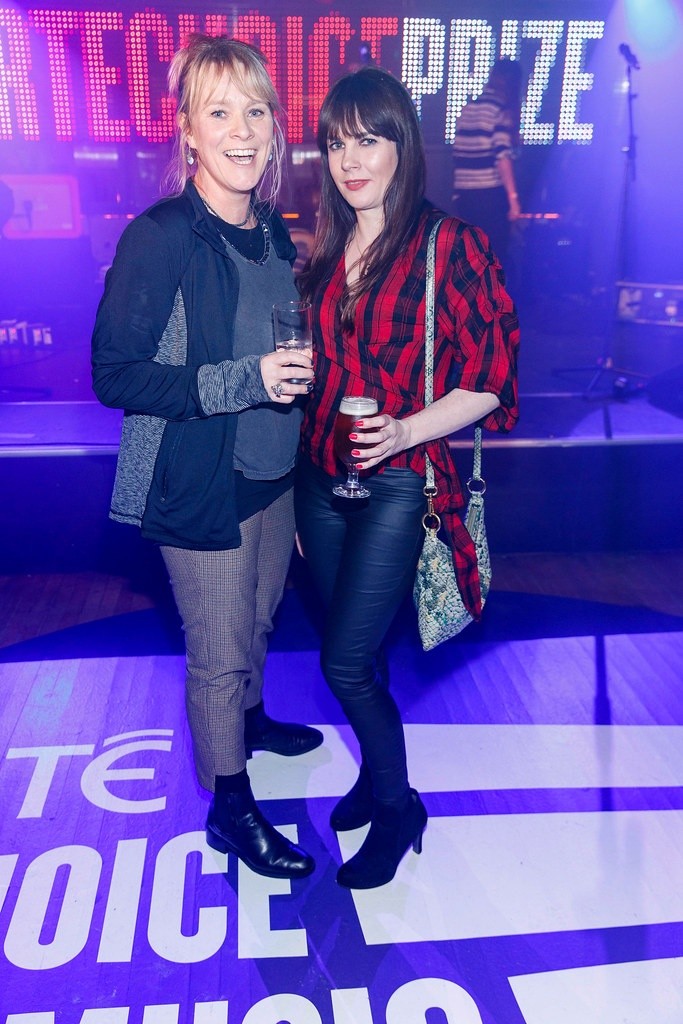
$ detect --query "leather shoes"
[205,789,316,879]
[244,700,324,760]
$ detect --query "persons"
[90,34,323,880]
[450,59,526,290]
[294,68,522,889]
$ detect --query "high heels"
[336,788,428,889]
[330,765,372,831]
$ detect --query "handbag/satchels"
[413,496,491,652]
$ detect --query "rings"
[271,382,284,397]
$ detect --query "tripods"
[552,64,650,404]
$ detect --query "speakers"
[0,172,81,239]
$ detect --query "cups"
[273,301,313,392]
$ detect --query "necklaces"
[354,230,372,264]
[201,198,252,228]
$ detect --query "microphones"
[619,42,640,70]
[24,200,32,222]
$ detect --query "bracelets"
[508,191,518,199]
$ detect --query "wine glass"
[331,396,379,498]
